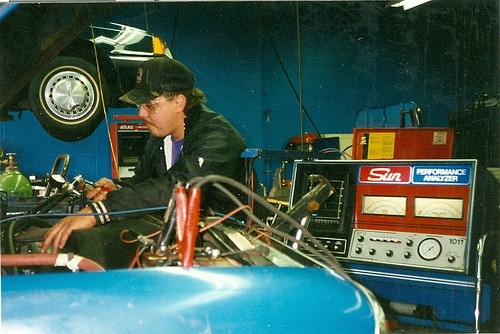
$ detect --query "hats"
[118,58,195,105]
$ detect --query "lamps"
[385,0,432,11]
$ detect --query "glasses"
[136,97,172,113]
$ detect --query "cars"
[0,0,114,145]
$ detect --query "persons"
[34,55,268,255]
